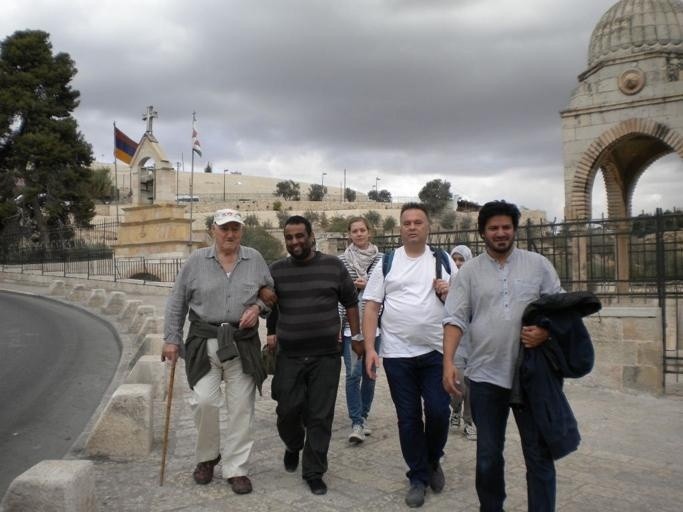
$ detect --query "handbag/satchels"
[260,341,280,374]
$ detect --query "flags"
[113,127,139,165]
[192,113,202,157]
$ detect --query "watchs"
[351,333,364,342]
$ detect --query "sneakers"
[308,478,326,494]
[193,454,221,483]
[285,450,299,471]
[406,464,444,507]
[349,416,373,441]
[228,476,253,493]
[451,403,478,440]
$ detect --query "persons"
[362,203,459,508]
[267,305,278,350]
[443,200,568,512]
[162,209,275,494]
[338,216,387,443]
[450,244,478,441]
[258,216,365,495]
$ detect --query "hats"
[214,210,245,226]
